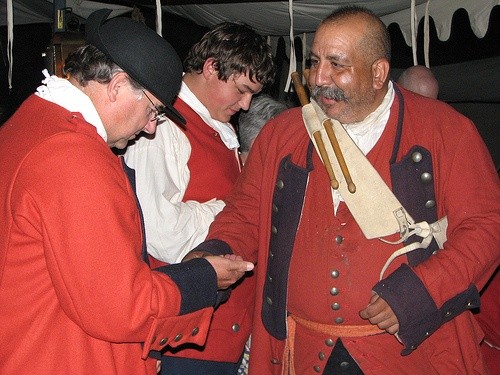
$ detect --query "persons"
[397,65,439,101]
[181,5,500,375]
[119,20,278,375]
[0,8,254,375]
[237,95,289,375]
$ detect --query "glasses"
[135,80,166,124]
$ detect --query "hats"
[82,8,188,126]
